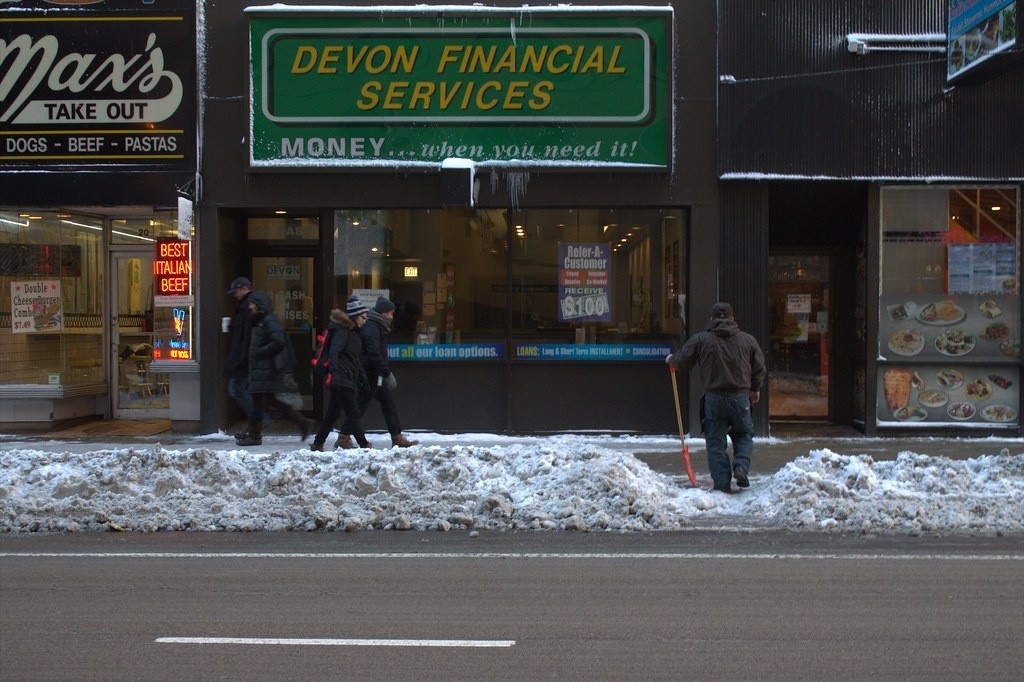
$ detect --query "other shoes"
[713,484,731,493]
[234,431,247,438]
[236,436,262,446]
[334,433,359,449]
[734,465,749,487]
[301,417,315,442]
[361,443,372,448]
[309,444,324,452]
[392,434,418,447]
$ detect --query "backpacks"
[311,327,339,376]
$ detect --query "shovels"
[669,362,697,488]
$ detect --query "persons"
[335,297,421,449]
[227,278,271,439]
[666,303,766,492]
[309,295,375,452]
[236,290,314,446]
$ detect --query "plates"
[892,406,928,422]
[918,390,949,408]
[934,330,977,357]
[935,371,964,390]
[915,303,965,327]
[980,404,1017,423]
[967,381,991,401]
[888,328,925,357]
[947,401,977,420]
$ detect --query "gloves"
[384,372,397,392]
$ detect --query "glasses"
[360,314,368,321]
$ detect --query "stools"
[65,356,170,406]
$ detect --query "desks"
[25,330,153,386]
[768,332,821,377]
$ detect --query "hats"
[345,295,369,316]
[227,277,251,294]
[713,303,733,317]
[375,296,395,313]
